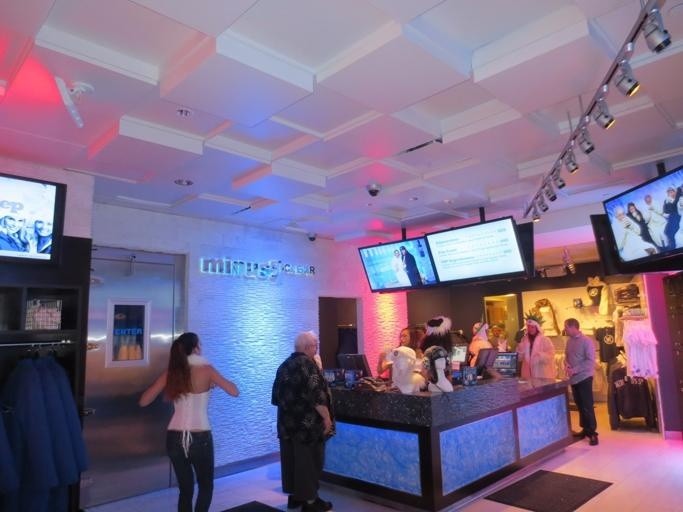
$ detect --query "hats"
[422,344,449,384]
[524,319,542,336]
[391,346,416,361]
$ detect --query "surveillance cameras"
[367,185,380,198]
[308,234,317,241]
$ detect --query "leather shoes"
[572,429,586,438]
[589,433,598,446]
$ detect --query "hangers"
[30,341,61,355]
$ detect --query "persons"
[563,318,598,446]
[0,210,31,254]
[626,202,663,255]
[515,309,555,379]
[400,246,423,286]
[29,217,54,254]
[468,321,494,368]
[642,194,670,248]
[674,185,683,249]
[137,332,241,512]
[663,187,681,250]
[611,206,658,262]
[416,316,455,393]
[391,250,412,287]
[487,323,513,353]
[270,330,338,512]
[376,326,430,393]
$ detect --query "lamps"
[522,1,681,222]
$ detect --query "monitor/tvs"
[516,221,534,279]
[446,342,469,365]
[491,352,518,377]
[590,214,625,277]
[338,354,372,377]
[601,165,683,266]
[0,173,68,266]
[475,348,497,373]
[425,217,527,285]
[357,236,437,293]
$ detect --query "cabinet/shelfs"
[0,281,81,511]
